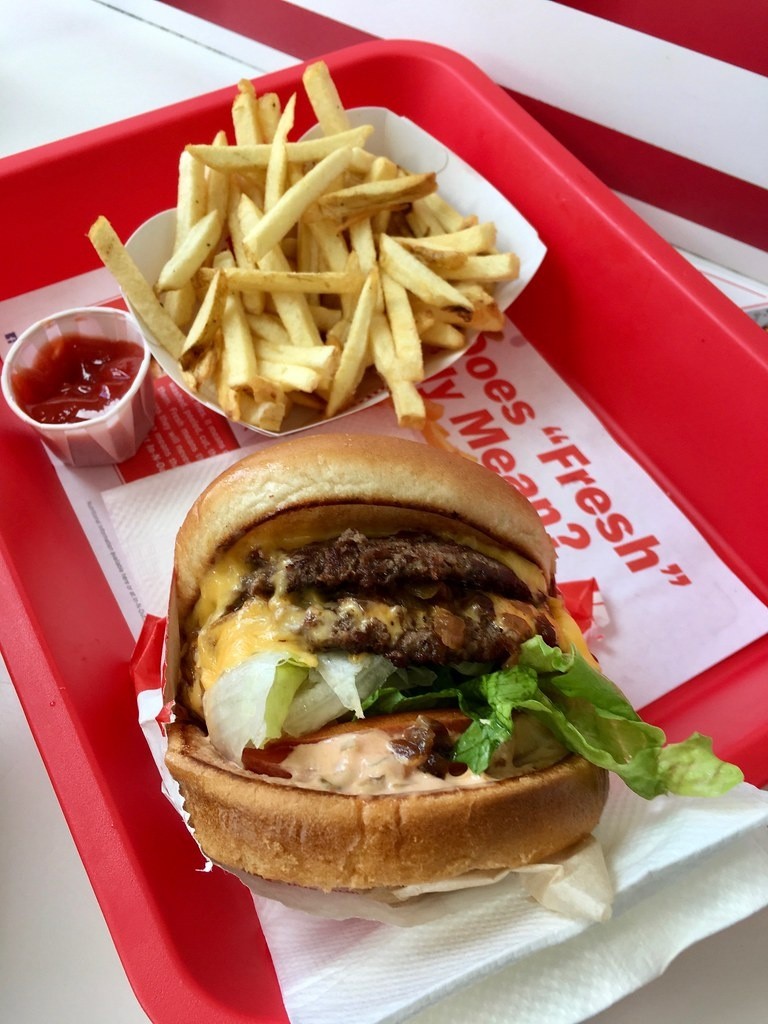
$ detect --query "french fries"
[84,62,520,427]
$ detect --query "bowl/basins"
[2,308,155,468]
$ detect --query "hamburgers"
[156,432,742,894]
